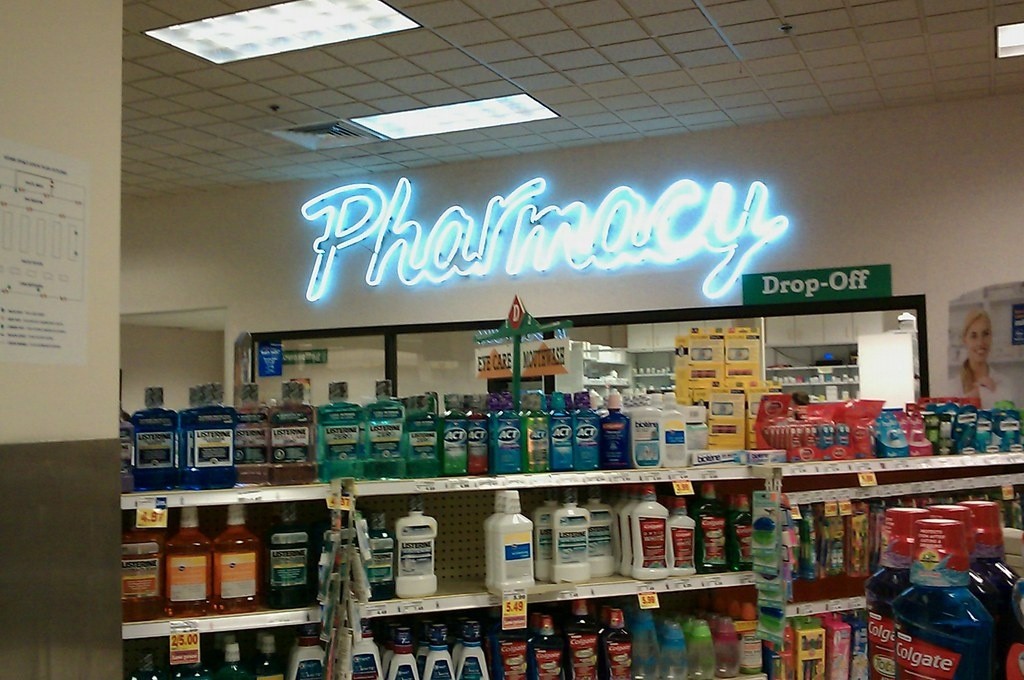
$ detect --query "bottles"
[120,381,763,680]
[864,501,1024,680]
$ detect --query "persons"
[947,308,1014,410]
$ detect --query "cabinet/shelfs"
[763,312,883,404]
[120,465,769,680]
[626,319,735,393]
[555,339,629,393]
[761,452,1024,617]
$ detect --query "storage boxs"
[724,327,761,388]
[674,327,725,389]
[763,608,869,680]
[745,386,783,450]
[692,389,746,450]
